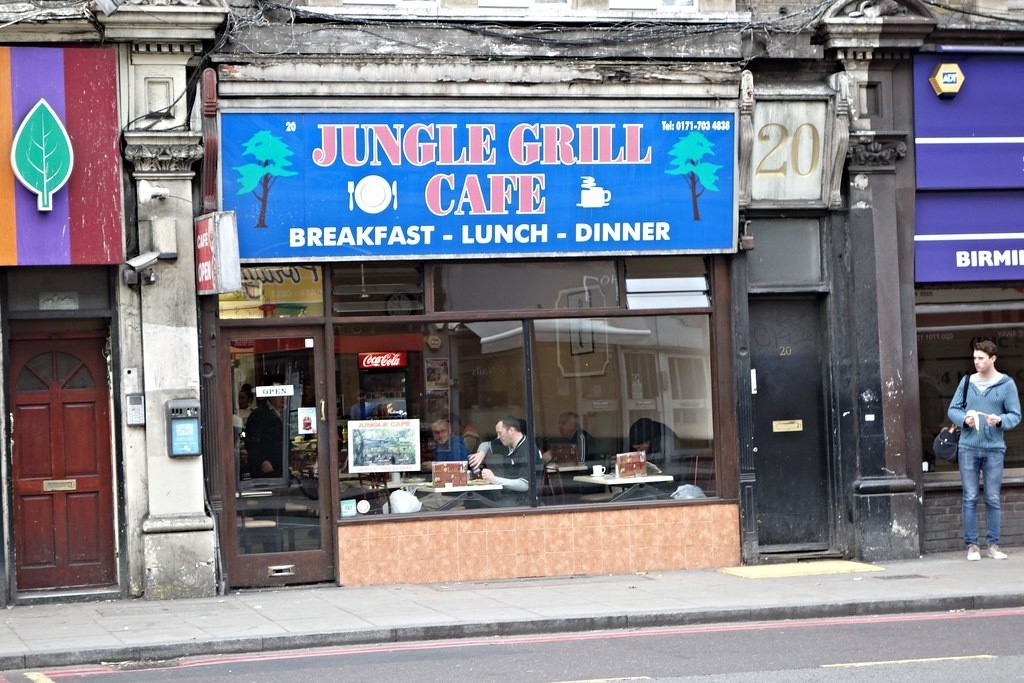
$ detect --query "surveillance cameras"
[125,252,160,272]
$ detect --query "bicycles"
[297,448,323,501]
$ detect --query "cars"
[349,398,408,419]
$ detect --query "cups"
[592,465,606,474]
[391,473,401,483]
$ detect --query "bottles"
[341,426,348,440]
[467,454,481,478]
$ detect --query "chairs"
[237,436,716,553]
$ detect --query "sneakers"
[966,543,981,560]
[987,544,1008,559]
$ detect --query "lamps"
[139,181,169,204]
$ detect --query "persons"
[629,418,683,493]
[422,420,468,474]
[948,341,1022,561]
[351,390,373,419]
[468,416,544,506]
[558,412,600,462]
[244,378,285,553]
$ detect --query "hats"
[630,417,657,445]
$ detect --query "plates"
[590,474,605,477]
[468,481,488,485]
[403,478,426,483]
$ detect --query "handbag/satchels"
[933,427,962,463]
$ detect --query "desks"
[573,473,674,503]
[416,486,505,511]
[548,463,588,473]
